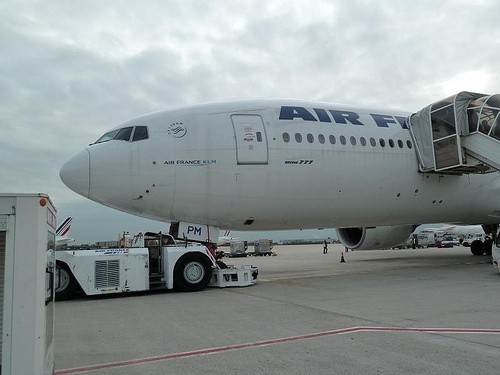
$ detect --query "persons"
[323,240,327,254]
[411,235,416,249]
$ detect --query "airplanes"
[59,90,500,255]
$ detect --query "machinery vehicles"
[55,230,257,300]
